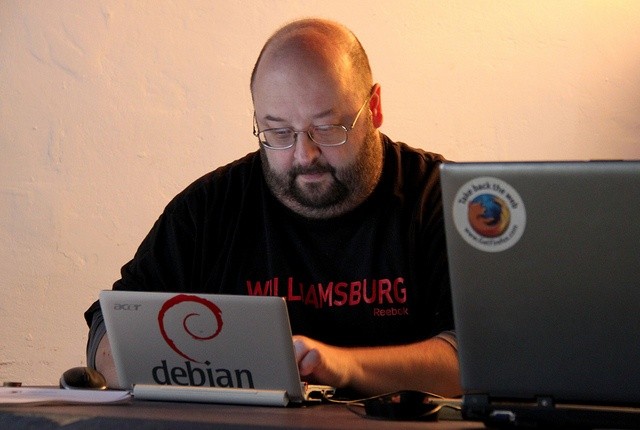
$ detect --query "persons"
[84,17,466,399]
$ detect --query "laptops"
[440,160,640,430]
[99,290,336,405]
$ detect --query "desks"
[0,385,485,430]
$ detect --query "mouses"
[59,367,106,391]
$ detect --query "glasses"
[252,93,371,150]
